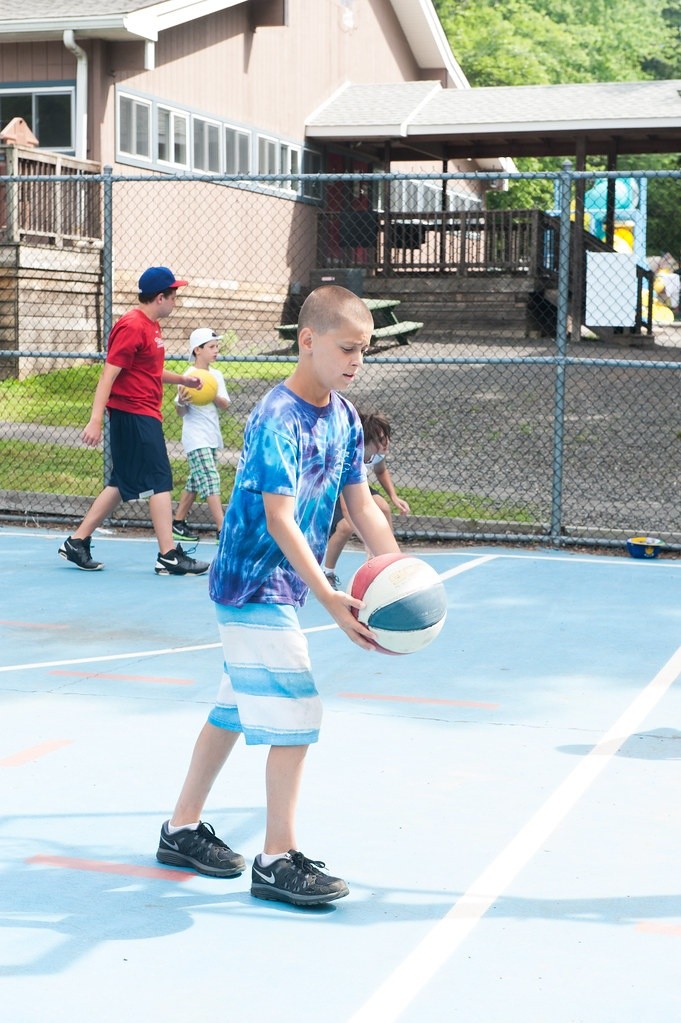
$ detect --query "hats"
[189,327,224,362]
[138,266,187,295]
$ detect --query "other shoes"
[324,569,337,591]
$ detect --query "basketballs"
[180,368,218,407]
[347,551,449,656]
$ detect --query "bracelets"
[174,395,186,408]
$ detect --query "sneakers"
[251,851,349,906]
[155,543,211,576]
[58,535,104,571]
[216,532,221,545]
[157,818,246,878]
[172,521,199,542]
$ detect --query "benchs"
[273,324,300,353]
[370,321,422,351]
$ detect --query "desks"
[361,298,407,350]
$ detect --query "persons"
[157,286,402,907]
[324,410,412,592]
[173,327,232,540]
[57,266,211,579]
